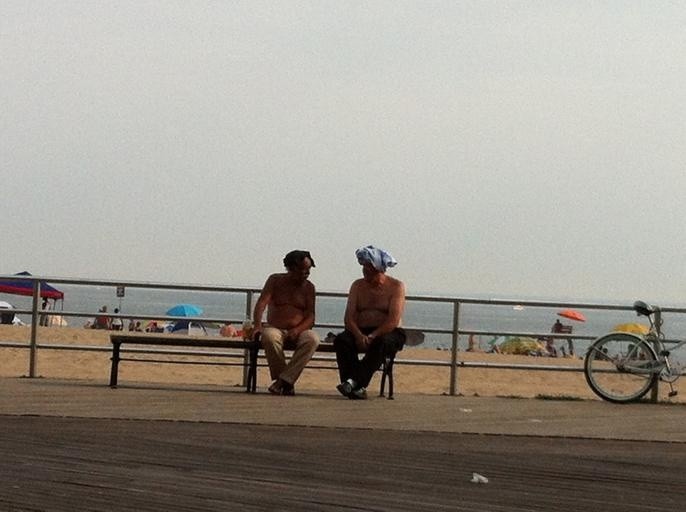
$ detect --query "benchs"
[105,333,400,401]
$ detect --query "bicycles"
[582,299,685,404]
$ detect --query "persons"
[40,297,51,326]
[466,335,645,361]
[85,306,175,332]
[334,246,407,399]
[552,319,562,333]
[221,322,237,337]
[248,250,318,395]
[324,332,336,351]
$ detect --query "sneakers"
[268,379,295,395]
[337,381,368,399]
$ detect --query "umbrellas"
[613,322,651,335]
[167,303,202,317]
[404,330,424,347]
[557,310,586,326]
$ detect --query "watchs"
[368,334,375,339]
